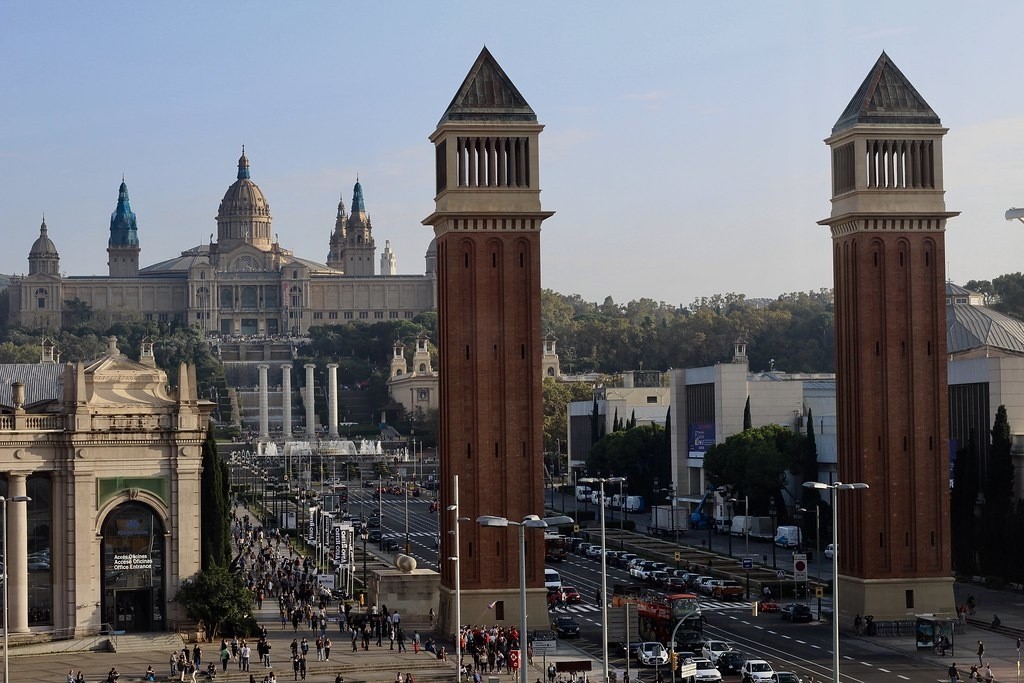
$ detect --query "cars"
[684,657,722,683]
[700,640,732,665]
[576,485,644,514]
[635,642,669,667]
[544,528,745,602]
[263,468,440,552]
[615,635,644,659]
[556,585,580,605]
[824,543,839,559]
[673,651,699,678]
[716,651,746,676]
[769,671,800,683]
[740,659,775,683]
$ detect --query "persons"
[548,590,572,612]
[956,596,975,623]
[338,602,535,683]
[948,663,960,683]
[146,666,155,681]
[235,423,299,442]
[1016,638,1021,657]
[109,668,120,683]
[929,636,949,656]
[229,498,332,637]
[969,664,993,683]
[223,332,295,342]
[537,663,585,683]
[854,614,877,637]
[623,672,629,683]
[66,670,85,683]
[169,624,342,683]
[977,641,984,667]
[992,615,1000,628]
[596,588,603,607]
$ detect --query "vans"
[544,569,563,594]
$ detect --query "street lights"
[476,514,575,683]
[803,481,869,683]
[0,496,32,683]
[578,476,625,683]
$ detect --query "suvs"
[551,615,581,639]
[675,629,706,651]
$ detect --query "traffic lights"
[673,653,678,671]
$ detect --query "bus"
[635,592,707,648]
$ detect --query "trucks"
[689,487,802,549]
[647,505,689,538]
